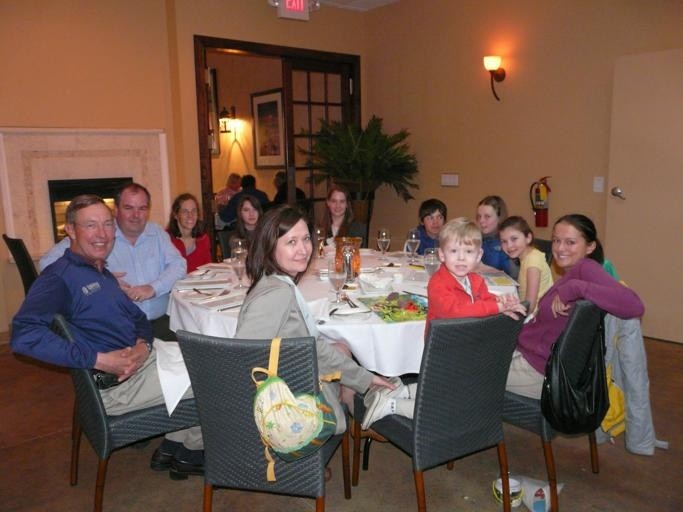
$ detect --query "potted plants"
[295,116,421,202]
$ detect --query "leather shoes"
[151,448,173,470]
[168,456,204,480]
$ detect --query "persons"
[9,194,207,482]
[503,214,645,398]
[38,183,187,340]
[233,202,397,482]
[167,173,367,271]
[359,218,527,430]
[408,195,554,317]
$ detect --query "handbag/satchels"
[541,352,611,433]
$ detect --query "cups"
[314,255,330,281]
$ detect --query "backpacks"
[253,337,336,464]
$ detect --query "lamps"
[482,54,505,103]
[216,107,236,135]
[267,1,321,22]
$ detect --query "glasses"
[73,220,116,233]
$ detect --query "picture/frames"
[248,86,285,170]
[204,69,221,157]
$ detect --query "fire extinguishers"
[530,176,552,227]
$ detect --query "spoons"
[342,293,357,308]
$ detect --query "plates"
[175,253,244,313]
[326,297,371,317]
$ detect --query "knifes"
[402,290,429,301]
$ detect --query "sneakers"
[360,392,386,431]
[363,377,404,408]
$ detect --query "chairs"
[170,328,353,512]
[359,300,531,512]
[1,233,41,301]
[45,314,203,512]
[513,235,553,270]
[503,296,614,512]
[215,230,232,260]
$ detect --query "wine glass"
[314,227,325,259]
[406,233,420,262]
[326,258,347,305]
[230,238,247,290]
[423,248,440,290]
[376,232,390,261]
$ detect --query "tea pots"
[333,236,361,282]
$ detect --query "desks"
[164,247,521,426]
